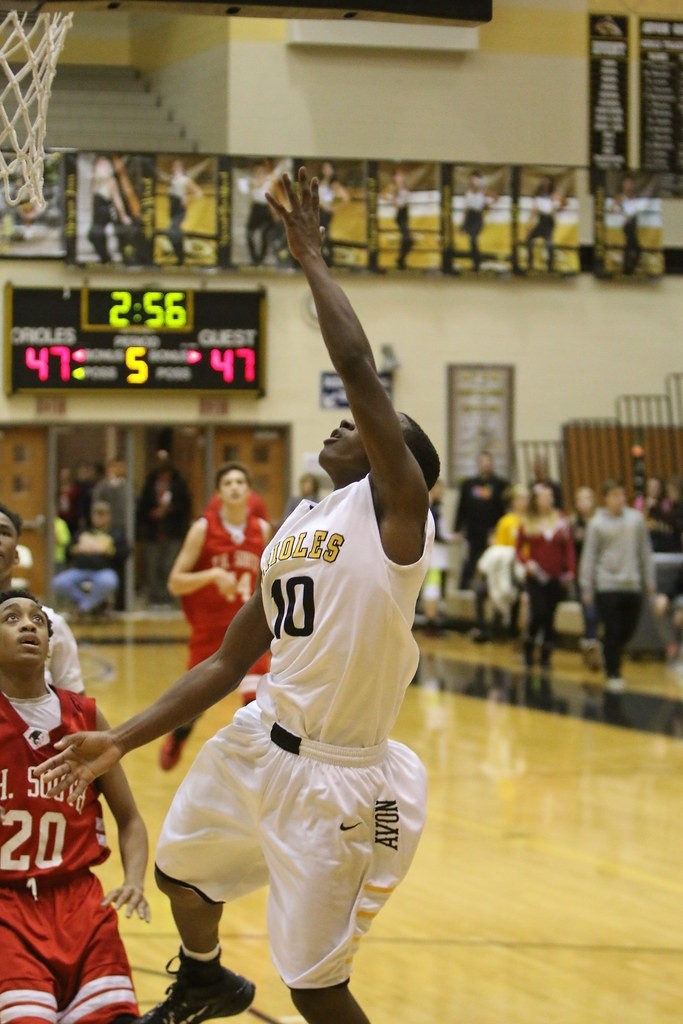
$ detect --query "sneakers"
[133,946,256,1024]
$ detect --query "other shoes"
[607,677,623,694]
[524,643,536,666]
[160,728,191,770]
[541,647,552,668]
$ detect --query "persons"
[156,159,203,265]
[423,451,683,694]
[612,175,659,275]
[318,162,350,265]
[283,474,320,518]
[0,589,151,1024]
[376,166,415,270]
[245,162,281,266]
[34,165,441,1024]
[459,170,499,271]
[45,446,195,621]
[524,182,563,274]
[161,462,271,769]
[87,155,132,263]
[0,504,84,697]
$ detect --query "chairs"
[447,549,683,658]
